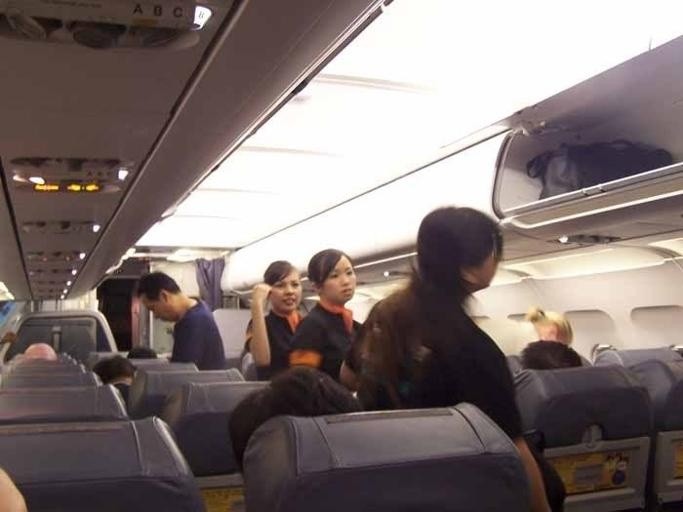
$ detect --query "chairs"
[0,348,683,512]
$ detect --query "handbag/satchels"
[538,139,673,201]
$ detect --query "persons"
[2,332,157,402]
[228,369,364,473]
[287,248,362,380]
[243,257,306,366]
[338,206,553,510]
[136,271,227,370]
[512,306,583,370]
